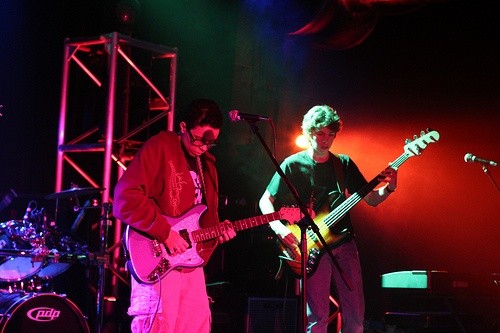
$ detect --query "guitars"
[276,128,440,280]
[124,203,305,284]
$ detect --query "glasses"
[186,127,219,149]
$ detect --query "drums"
[38,222,75,280]
[0,289,91,333]
[0,219,46,283]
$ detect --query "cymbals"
[48,187,107,198]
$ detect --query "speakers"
[245,297,299,333]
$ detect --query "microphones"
[464,153,498,166]
[228,110,270,122]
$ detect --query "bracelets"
[384,184,393,194]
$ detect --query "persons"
[258,105,399,333]
[112,100,236,332]
